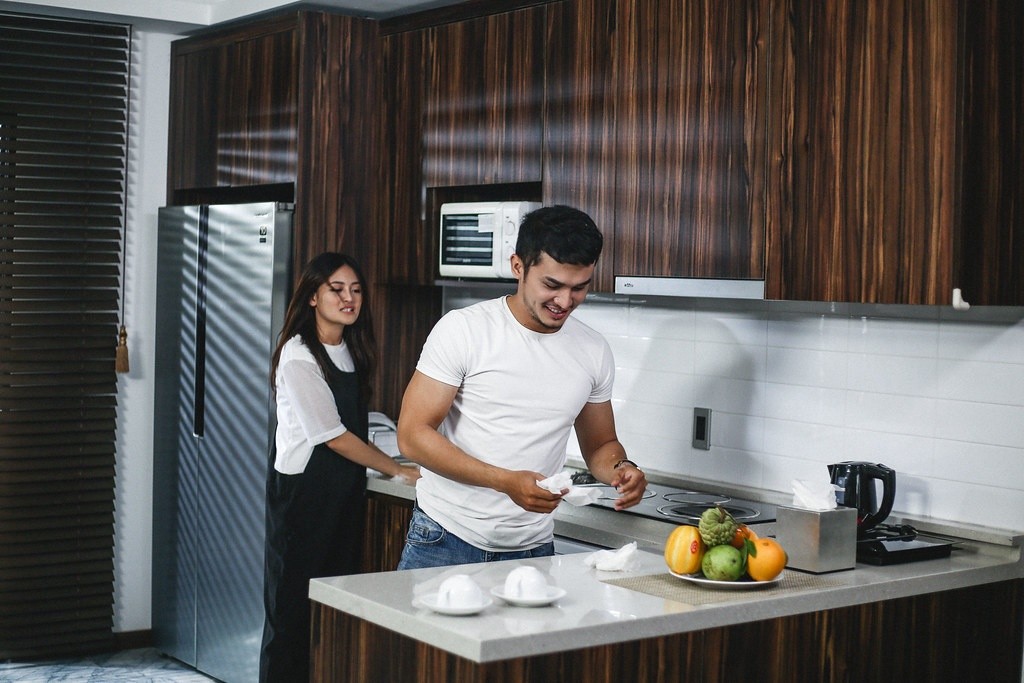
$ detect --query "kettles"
[826,461,897,533]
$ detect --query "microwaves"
[435,200,542,280]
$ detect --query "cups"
[505,566,548,600]
[437,574,485,609]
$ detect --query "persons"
[256,250,423,683]
[396,205,647,588]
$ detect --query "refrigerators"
[151,200,295,683]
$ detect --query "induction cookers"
[558,464,780,528]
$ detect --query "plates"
[420,592,493,616]
[668,566,784,592]
[491,583,568,608]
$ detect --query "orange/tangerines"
[728,526,785,581]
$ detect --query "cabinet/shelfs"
[168,6,380,189]
[383,1,1023,306]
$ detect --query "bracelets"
[612,460,642,492]
